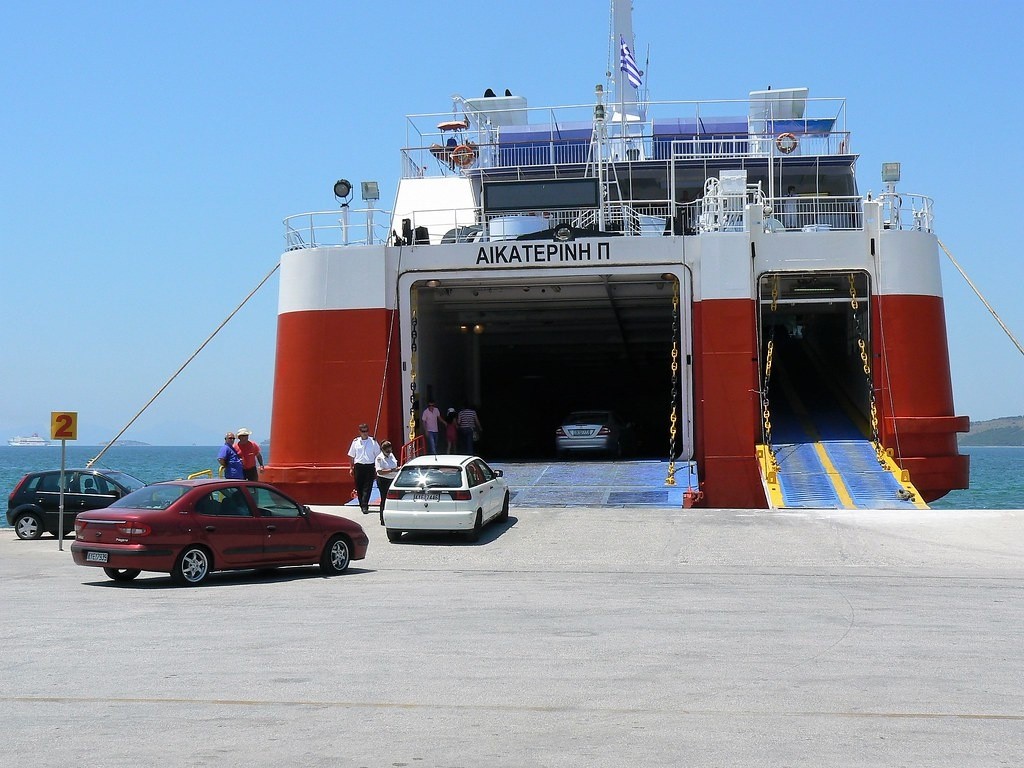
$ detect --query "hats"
[448,408,455,412]
[234,427,252,439]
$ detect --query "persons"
[421,399,448,456]
[232,428,265,508]
[781,185,800,228]
[217,432,244,494]
[375,439,401,526]
[444,407,458,455]
[456,400,483,456]
[346,423,382,514]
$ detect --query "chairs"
[84,479,98,493]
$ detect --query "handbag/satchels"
[472,430,479,441]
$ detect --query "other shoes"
[360,506,368,514]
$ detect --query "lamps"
[333,179,354,206]
[881,162,901,183]
[425,280,441,287]
[360,182,380,199]
[661,274,674,280]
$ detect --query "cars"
[6,467,147,540]
[70,478,369,586]
[554,410,622,460]
[383,455,509,541]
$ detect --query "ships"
[7,432,48,446]
[257,1,970,508]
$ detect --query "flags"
[619,36,642,89]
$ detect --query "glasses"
[428,405,434,407]
[240,435,248,436]
[360,429,368,432]
[228,437,235,440]
[384,449,392,453]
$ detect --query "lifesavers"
[776,133,797,153]
[453,145,473,165]
